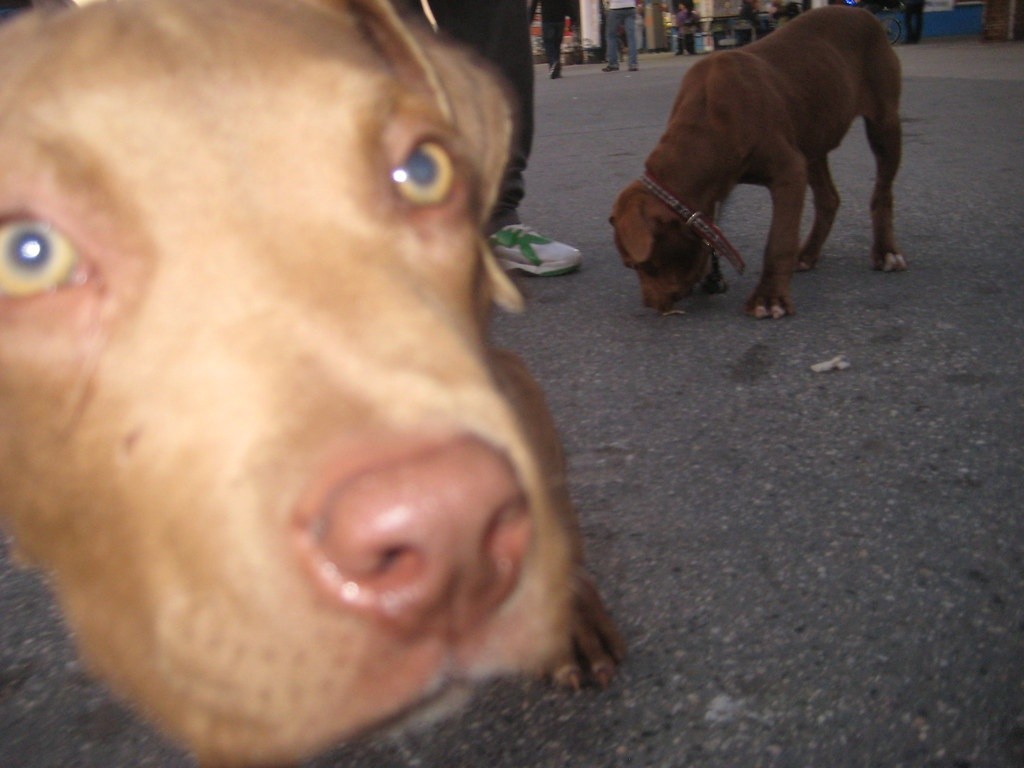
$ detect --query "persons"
[671,0,697,55]
[602,0,638,72]
[739,0,800,36]
[828,0,926,44]
[527,0,578,79]
[428,0,584,277]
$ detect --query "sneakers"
[490,223,582,276]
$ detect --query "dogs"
[0,0,631,767]
[606,5,903,321]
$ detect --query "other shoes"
[602,66,619,72]
[549,61,562,79]
[627,67,638,71]
